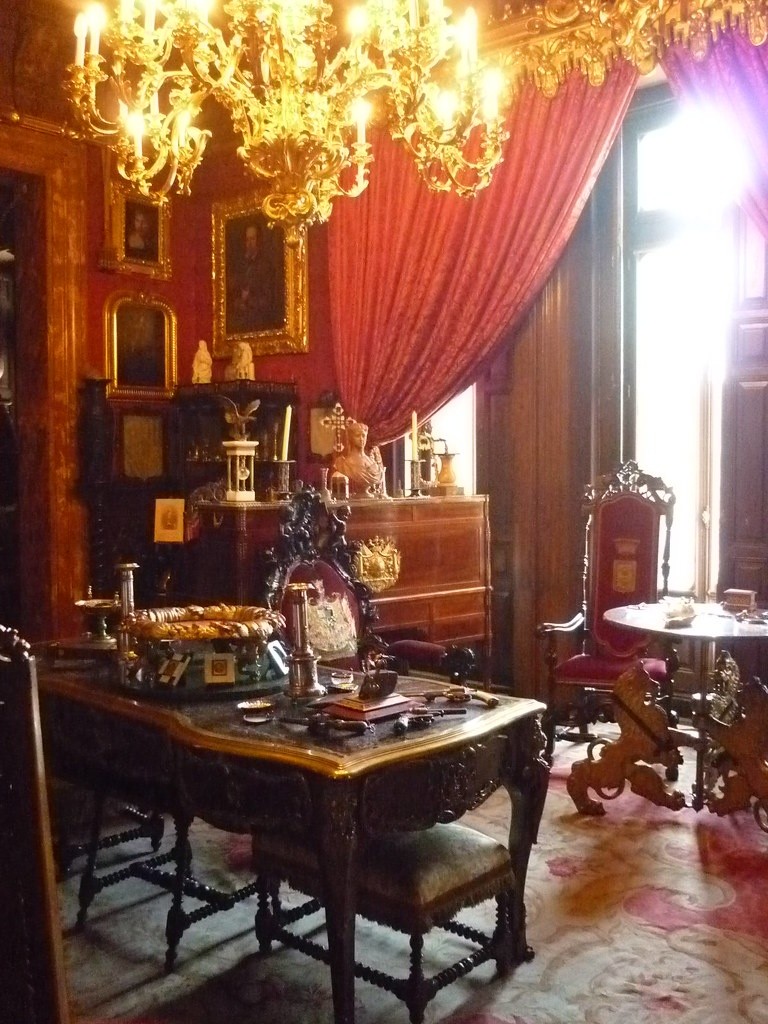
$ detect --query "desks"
[195,495,495,684]
[26,635,555,1024]
[602,600,768,814]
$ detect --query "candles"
[281,405,293,459]
[412,409,420,461]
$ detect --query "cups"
[331,671,353,685]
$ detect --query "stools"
[67,786,258,976]
[250,814,515,1024]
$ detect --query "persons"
[227,222,284,332]
[129,206,154,250]
[334,423,381,499]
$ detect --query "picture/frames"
[117,406,168,486]
[211,187,309,359]
[101,178,173,284]
[148,492,189,546]
[103,289,179,399]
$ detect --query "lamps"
[56,0,514,237]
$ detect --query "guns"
[394,707,467,736]
[278,713,368,737]
[402,683,500,707]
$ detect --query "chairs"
[260,485,482,689]
[534,458,683,781]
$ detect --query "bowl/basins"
[236,699,276,722]
